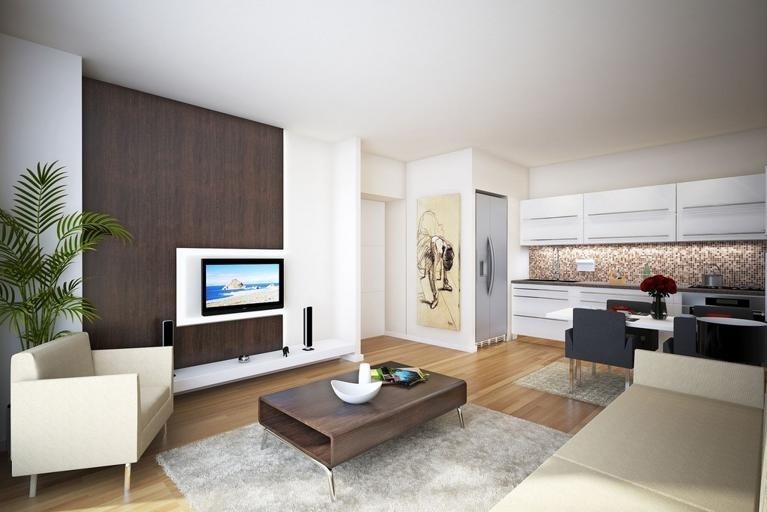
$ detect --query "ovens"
[681,291,764,320]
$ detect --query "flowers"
[640,275,678,302]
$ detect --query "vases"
[650,301,668,320]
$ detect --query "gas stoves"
[688,284,764,291]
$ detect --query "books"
[625,317,640,324]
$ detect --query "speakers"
[302,307,314,351]
[162,320,176,377]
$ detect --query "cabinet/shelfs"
[520,194,583,246]
[676,173,767,240]
[512,283,682,343]
[583,184,676,244]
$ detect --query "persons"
[427,234,456,309]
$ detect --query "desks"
[625,314,767,332]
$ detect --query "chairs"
[606,299,659,351]
[565,308,644,393]
[689,305,752,320]
[10,332,174,497]
[663,317,698,357]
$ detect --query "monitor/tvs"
[201,258,284,316]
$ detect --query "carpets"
[514,360,633,407]
[154,403,573,512]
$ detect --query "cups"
[616,310,631,319]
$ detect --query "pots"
[702,274,723,287]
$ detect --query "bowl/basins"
[329,377,383,404]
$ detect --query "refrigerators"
[476,193,508,345]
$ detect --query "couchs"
[488,349,767,512]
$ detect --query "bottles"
[358,362,372,383]
